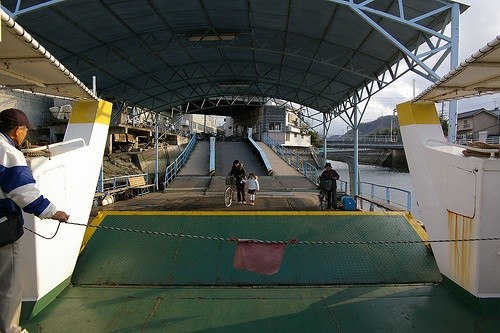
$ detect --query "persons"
[0,108,70,333]
[321,162,339,210]
[228,159,248,205]
[242,173,259,206]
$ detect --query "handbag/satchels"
[0,206,24,247]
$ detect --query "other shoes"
[239,200,254,206]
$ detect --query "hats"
[324,163,333,168]
[0,107,37,130]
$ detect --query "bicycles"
[224,174,237,208]
[315,176,337,211]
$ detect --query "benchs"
[128,176,155,199]
